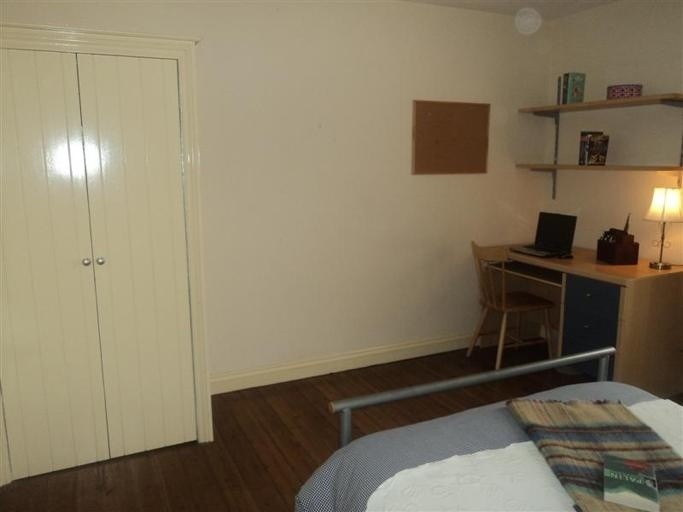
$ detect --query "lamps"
[645,187,683,270]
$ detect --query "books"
[603,454,661,511]
[557,76,564,106]
[563,72,586,105]
[578,131,609,166]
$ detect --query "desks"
[506,244,683,398]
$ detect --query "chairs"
[466,242,554,371]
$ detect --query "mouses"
[559,253,574,259]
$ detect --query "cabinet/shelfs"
[0,23,213,487]
[515,94,683,173]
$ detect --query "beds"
[294,347,683,512]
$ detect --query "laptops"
[510,212,577,258]
